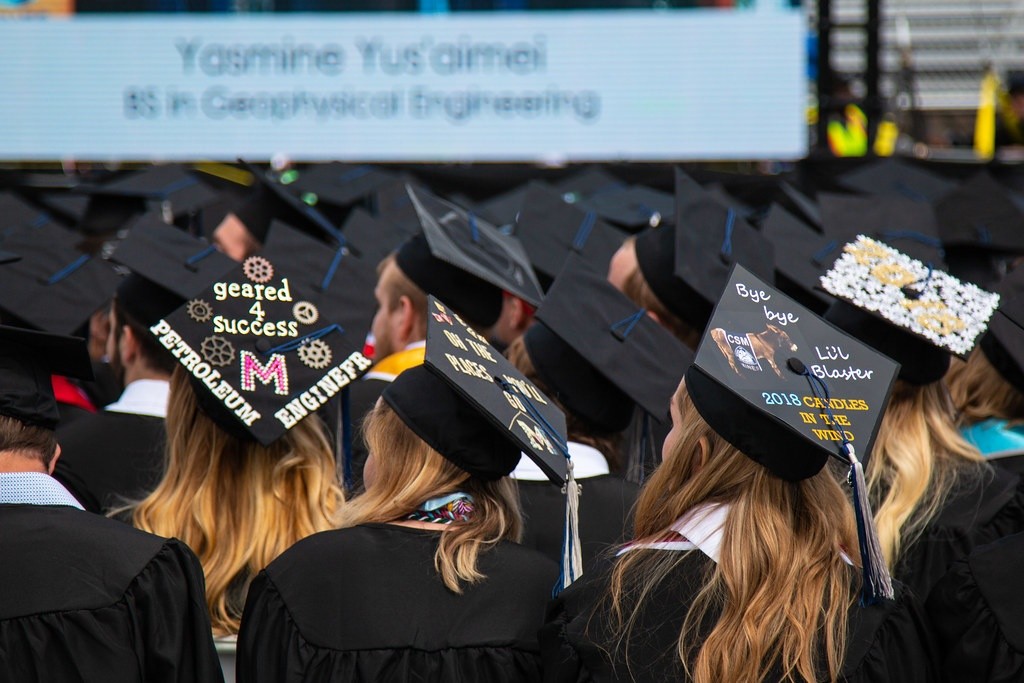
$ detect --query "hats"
[981,291,1023,390]
[0,325,95,428]
[685,261,901,603]
[382,293,583,589]
[532,249,695,428]
[147,243,375,448]
[810,228,1008,363]
[402,179,546,309]
[0,161,1024,290]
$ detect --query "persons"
[995,71,1024,147]
[0,162,1024,683]
